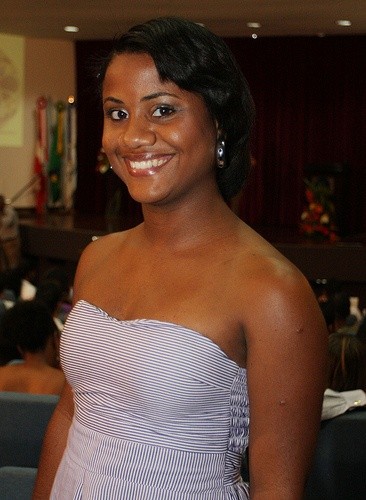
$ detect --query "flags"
[36,98,74,217]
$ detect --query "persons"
[0,194,68,366]
[326,334,366,392]
[31,17,325,500]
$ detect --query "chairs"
[0,390,366,500]
